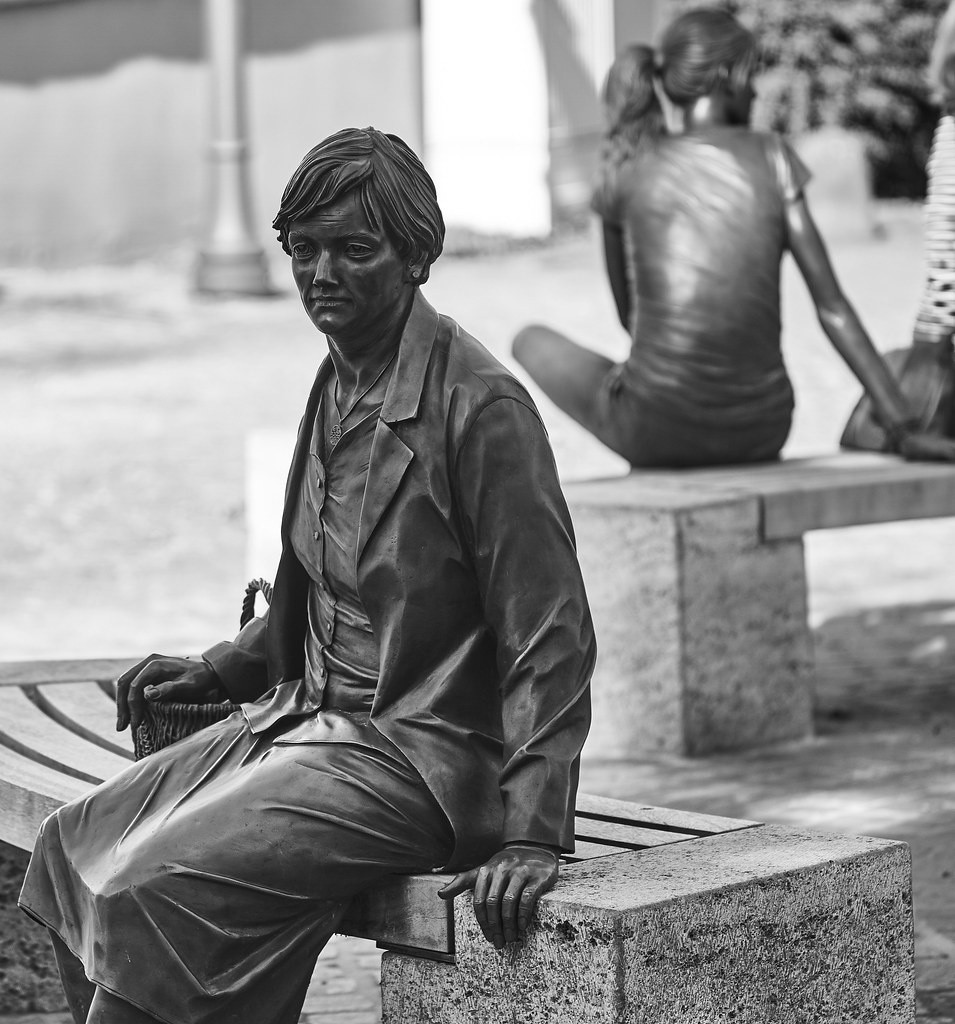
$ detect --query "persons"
[18,127,597,1024]
[511,9,955,469]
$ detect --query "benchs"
[1,656,917,1024]
[560,448,954,759]
